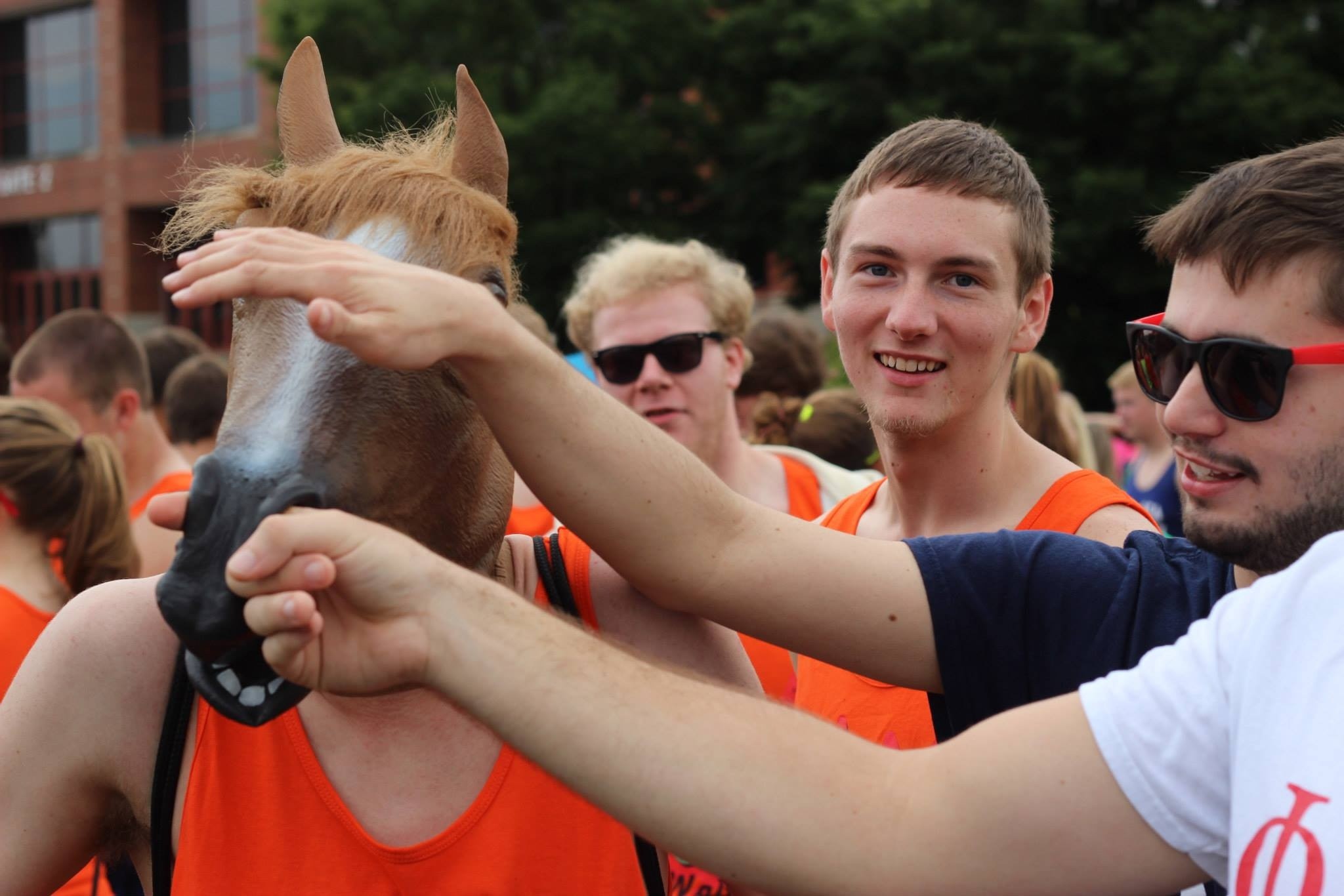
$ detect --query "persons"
[0,117,1344,896]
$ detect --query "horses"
[156,34,515,730]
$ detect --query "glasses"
[1124,311,1344,421]
[593,329,726,385]
